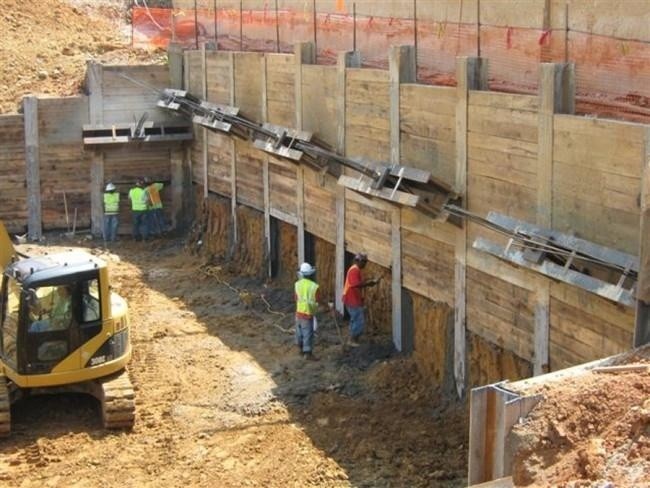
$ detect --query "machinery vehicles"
[0,220,136,440]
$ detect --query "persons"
[28,286,72,332]
[341,252,376,348]
[142,177,168,238]
[128,179,153,241]
[294,262,330,360]
[103,183,120,242]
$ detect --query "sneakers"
[346,340,361,347]
[304,353,321,359]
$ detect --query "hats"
[356,251,368,261]
[135,175,152,187]
[105,182,116,191]
[297,262,319,275]
